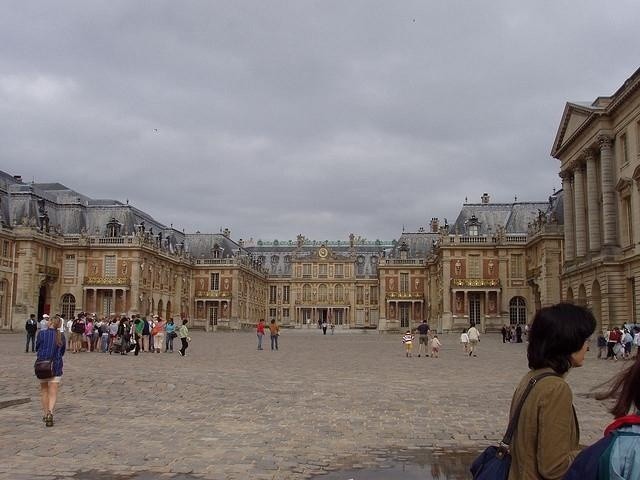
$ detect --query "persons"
[460,328,470,355]
[322,321,327,335]
[34,316,66,426]
[507,303,597,480]
[269,319,280,350]
[415,320,433,357]
[318,318,322,329]
[331,322,335,335]
[597,322,640,361]
[25,311,192,357]
[256,319,265,350]
[590,347,640,480]
[501,323,530,343]
[401,330,416,358]
[468,323,481,358]
[430,336,442,358]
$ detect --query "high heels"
[46,414,53,427]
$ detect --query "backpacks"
[567,430,640,480]
[75,323,81,332]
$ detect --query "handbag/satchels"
[171,332,177,338]
[113,338,122,346]
[470,446,511,480]
[34,359,55,378]
